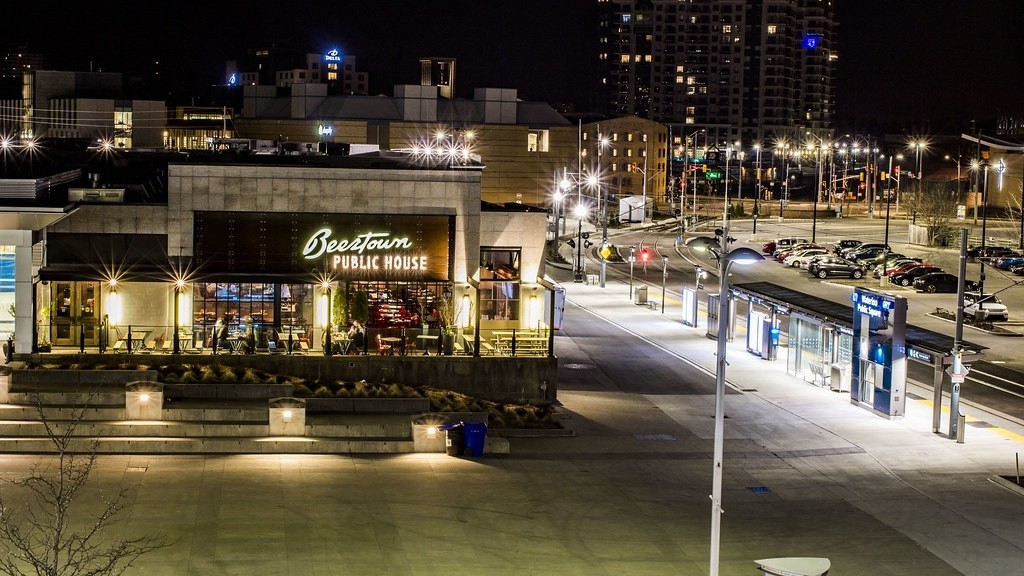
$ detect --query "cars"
[966,244,1024,276]
[762,239,1008,321]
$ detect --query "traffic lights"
[643,249,647,262]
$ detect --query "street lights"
[753,144,760,234]
[685,224,766,576]
[776,129,884,242]
[880,154,903,287]
[971,162,1006,323]
[662,254,669,312]
[630,246,636,300]
[685,130,706,212]
[694,264,702,328]
[909,140,925,225]
[550,119,609,283]
[945,145,960,204]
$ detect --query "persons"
[348,322,364,355]
[212,318,269,348]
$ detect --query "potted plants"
[462,300,476,353]
[37,308,51,352]
[439,297,458,356]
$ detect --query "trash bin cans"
[461,420,487,459]
[634,286,648,305]
[446,428,466,457]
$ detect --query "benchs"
[647,301,660,310]
[810,364,832,384]
[454,339,549,356]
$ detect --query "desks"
[336,338,354,356]
[501,338,550,354]
[462,335,485,355]
[228,338,247,355]
[492,332,539,348]
[416,335,438,356]
[279,333,299,355]
[179,339,192,354]
[380,337,401,355]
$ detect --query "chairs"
[114,326,418,355]
